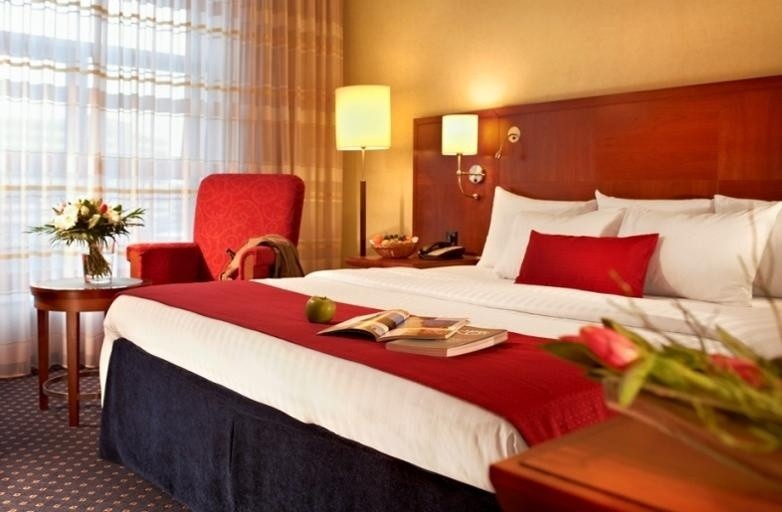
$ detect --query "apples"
[304,296,335,323]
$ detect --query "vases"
[81,238,117,286]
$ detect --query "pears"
[381,240,389,246]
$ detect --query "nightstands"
[343,252,481,270]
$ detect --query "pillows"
[712,194,780,300]
[617,202,781,302]
[494,207,630,283]
[476,187,600,269]
[515,230,661,298]
[595,189,717,237]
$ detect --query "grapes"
[384,232,406,240]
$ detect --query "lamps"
[333,82,394,257]
[439,112,488,200]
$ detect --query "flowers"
[20,195,145,277]
[535,310,780,463]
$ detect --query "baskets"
[368,235,421,259]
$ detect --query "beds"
[93,72,780,511]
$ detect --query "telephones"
[418,240,465,259]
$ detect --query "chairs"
[124,171,307,285]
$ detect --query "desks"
[30,277,142,428]
[486,407,780,512]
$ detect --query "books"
[384,321,509,356]
[319,309,468,341]
